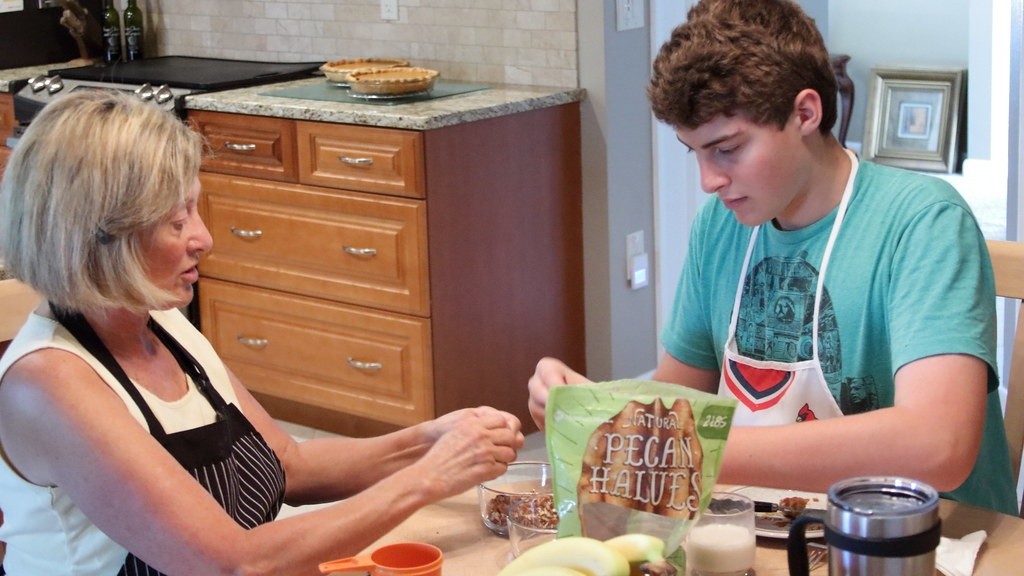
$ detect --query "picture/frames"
[860,68,967,174]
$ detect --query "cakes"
[319,58,410,83]
[344,68,440,94]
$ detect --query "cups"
[318,541,445,576]
[685,491,757,576]
[786,475,941,576]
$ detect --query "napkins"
[935,531,987,576]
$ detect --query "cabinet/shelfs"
[188,100,586,428]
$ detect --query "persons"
[0,91,525,575]
[528,1,1020,519]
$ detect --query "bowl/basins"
[506,495,576,560]
[477,461,556,538]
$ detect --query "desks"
[357,431,1024,576]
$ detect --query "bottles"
[101,0,122,66]
[123,0,143,62]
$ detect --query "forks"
[807,543,829,569]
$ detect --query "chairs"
[984,238,1024,491]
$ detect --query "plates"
[697,484,828,539]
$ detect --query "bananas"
[495,533,677,576]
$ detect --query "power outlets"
[626,230,645,281]
[615,0,645,31]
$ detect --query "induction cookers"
[13,55,326,133]
